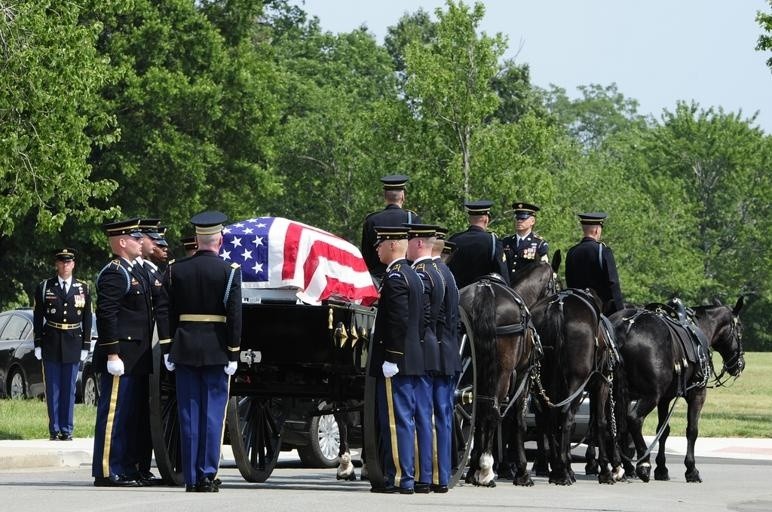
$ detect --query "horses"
[605,294,746,483]
[527,287,619,486]
[457,245,562,488]
[332,399,370,481]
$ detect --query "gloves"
[224,361,238,376]
[34,347,41,360]
[80,350,89,361]
[163,354,175,371]
[381,361,399,379]
[106,358,125,377]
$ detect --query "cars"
[0,311,102,408]
[220,390,361,467]
[520,382,636,447]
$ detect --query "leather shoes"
[187,484,199,491]
[93,470,169,487]
[49,431,60,441]
[198,481,218,492]
[365,480,449,493]
[61,434,72,440]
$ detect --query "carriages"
[147,218,757,488]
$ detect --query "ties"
[519,239,522,247]
[62,281,66,296]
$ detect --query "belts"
[46,320,81,330]
[179,314,226,323]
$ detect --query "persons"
[32,247,92,441]
[565,213,624,317]
[443,200,511,290]
[401,223,444,494]
[93,219,169,488]
[430,228,463,493]
[501,202,550,288]
[181,237,198,256]
[156,212,243,493]
[368,226,425,494]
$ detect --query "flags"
[219,217,379,306]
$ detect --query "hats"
[466,201,493,219]
[380,176,408,192]
[54,248,78,262]
[578,214,608,229]
[512,203,540,219]
[373,221,457,256]
[107,210,228,251]
